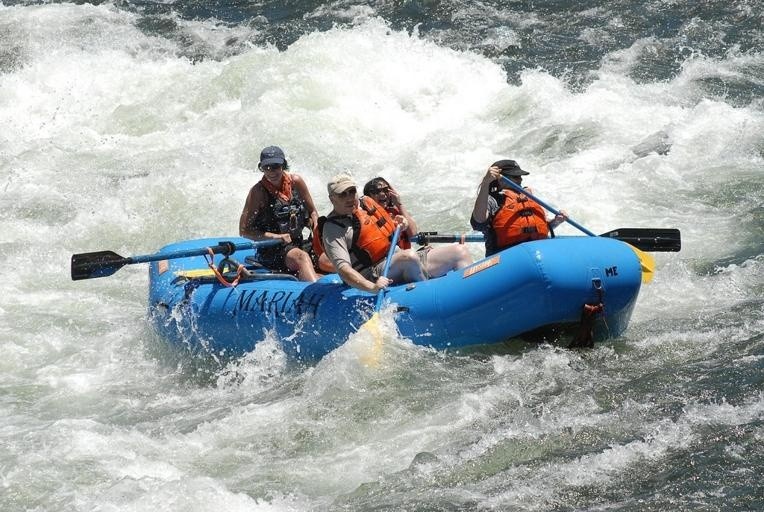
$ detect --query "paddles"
[411,228,681,251]
[174,264,267,278]
[71,239,286,281]
[497,174,655,284]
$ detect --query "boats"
[144,231,645,365]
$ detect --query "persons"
[363,177,418,251]
[238,146,337,283]
[322,175,475,294]
[469,159,569,258]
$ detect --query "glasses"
[370,187,388,194]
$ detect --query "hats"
[328,175,357,194]
[260,146,284,168]
[492,160,529,176]
